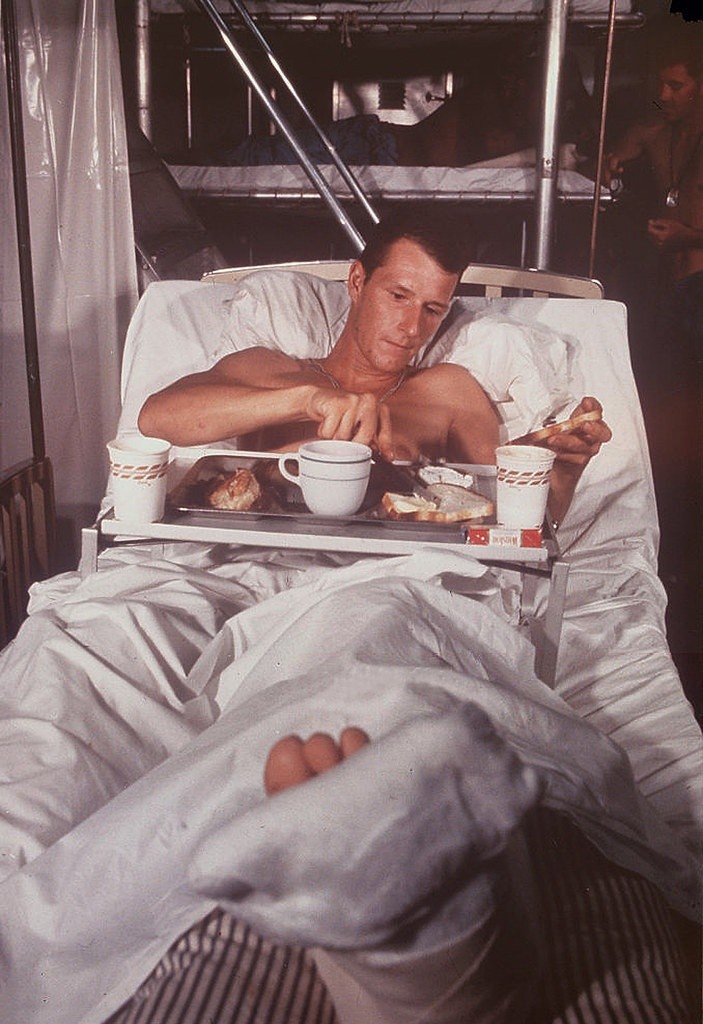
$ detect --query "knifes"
[354,427,442,507]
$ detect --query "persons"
[197,64,592,167]
[596,44,703,350]
[137,209,612,798]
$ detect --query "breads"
[501,410,603,446]
[210,468,260,510]
[381,482,494,523]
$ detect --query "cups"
[106,436,173,522]
[495,445,556,534]
[279,440,373,515]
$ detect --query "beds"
[0,258,703,1024]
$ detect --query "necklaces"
[665,127,703,208]
[306,358,406,402]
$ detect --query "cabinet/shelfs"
[136,0,646,302]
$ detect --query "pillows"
[208,268,569,449]
